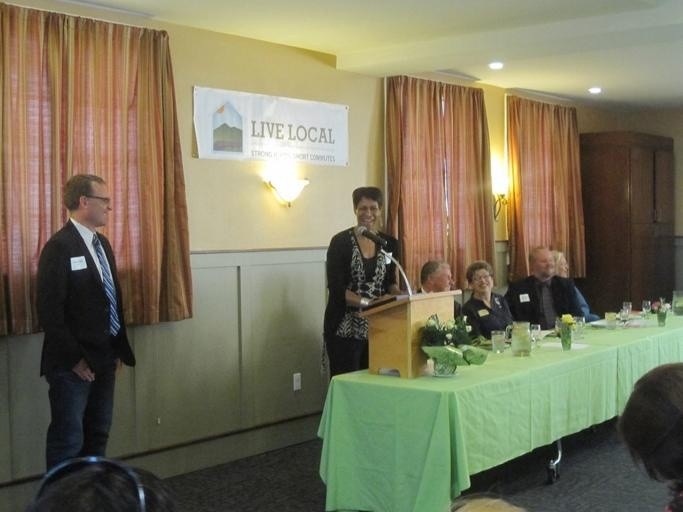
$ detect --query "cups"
[490,331,504,354]
[555,291,682,338]
[531,324,541,344]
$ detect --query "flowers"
[659,297,671,313]
[419,314,488,367]
[561,313,577,327]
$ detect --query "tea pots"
[505,321,530,357]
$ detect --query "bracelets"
[360,297,370,310]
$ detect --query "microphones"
[357,226,387,247]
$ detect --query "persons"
[36,174,135,476]
[620,363,683,512]
[416,261,461,319]
[450,498,524,512]
[503,247,590,330]
[26,463,176,512]
[552,251,599,322]
[323,187,401,378]
[461,263,514,341]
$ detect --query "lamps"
[268,178,310,207]
[489,165,508,220]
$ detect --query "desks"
[316,301,683,512]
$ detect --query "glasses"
[85,193,111,208]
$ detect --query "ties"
[91,234,121,337]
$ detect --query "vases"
[559,325,571,351]
[657,310,667,327]
[432,358,457,377]
